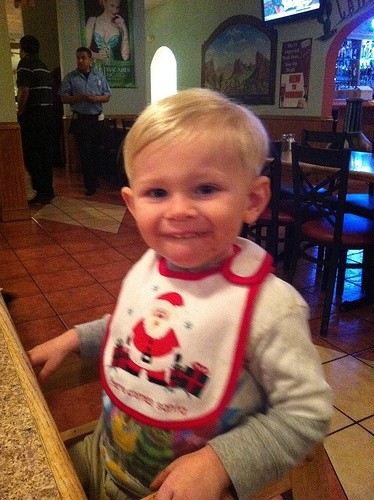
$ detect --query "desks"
[263,150,374,203]
[0,294,85,499]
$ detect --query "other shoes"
[28,191,55,205]
[84,188,96,195]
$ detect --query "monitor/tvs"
[261,0,325,27]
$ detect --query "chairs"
[60,418,348,500]
[239,131,374,337]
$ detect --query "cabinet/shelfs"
[334,39,362,89]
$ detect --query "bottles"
[360,62,374,88]
[282,133,295,164]
[335,61,357,90]
[338,41,358,59]
[362,41,374,59]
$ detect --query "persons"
[24,86,334,500]
[17,35,56,205]
[86,0,129,61]
[57,47,112,195]
